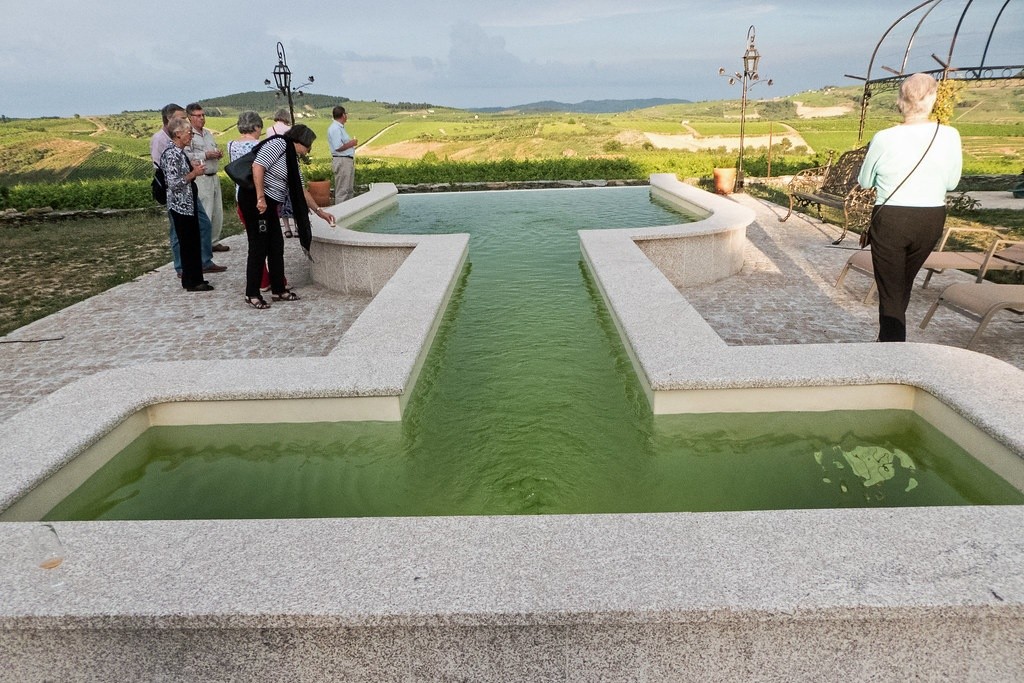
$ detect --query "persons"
[328,106,358,205]
[160,118,214,292]
[266,109,299,238]
[227,111,287,292]
[238,123,335,309]
[150,103,228,278]
[183,103,230,252]
[857,73,962,342]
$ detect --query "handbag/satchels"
[151,147,172,205]
[224,135,287,189]
[859,224,871,249]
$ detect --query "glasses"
[192,113,205,118]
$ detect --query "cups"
[213,147,219,153]
[352,136,357,141]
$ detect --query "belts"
[333,155,354,159]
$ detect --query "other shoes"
[182,281,213,291]
[203,261,227,273]
[259,277,294,291]
[176,271,182,277]
[212,244,229,252]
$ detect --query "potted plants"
[302,169,331,207]
[712,157,736,193]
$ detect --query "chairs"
[834,226,1024,303]
[918,282,1024,348]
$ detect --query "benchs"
[778,140,882,244]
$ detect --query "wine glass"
[329,219,337,235]
[198,159,207,176]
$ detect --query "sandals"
[294,231,300,237]
[245,295,271,309]
[271,290,301,301]
[284,231,293,238]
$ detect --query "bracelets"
[316,208,321,211]
[258,195,264,200]
